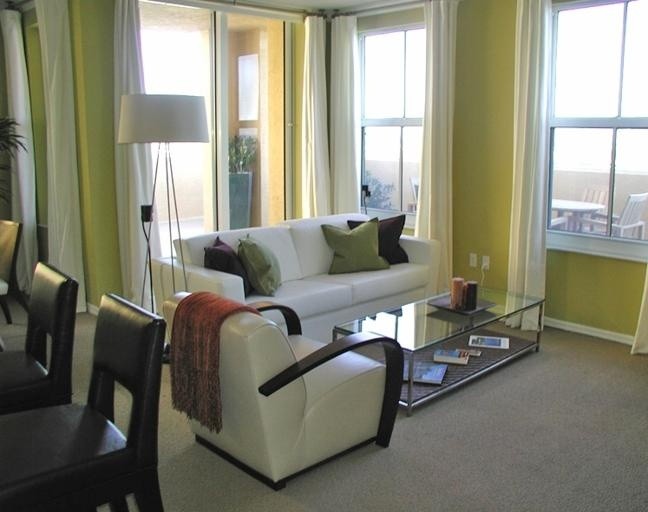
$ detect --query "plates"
[427,291,496,317]
[424,308,497,326]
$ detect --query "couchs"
[162,289,405,492]
[148,210,441,345]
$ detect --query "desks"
[550,199,606,233]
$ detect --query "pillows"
[201,234,252,297]
[347,214,410,265]
[235,234,282,297]
[320,218,392,275]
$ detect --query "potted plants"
[229,135,257,229]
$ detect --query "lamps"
[362,185,371,214]
[115,95,211,358]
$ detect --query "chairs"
[0,219,28,321]
[0,259,79,411]
[581,186,614,206]
[581,192,647,240]
[0,290,166,512]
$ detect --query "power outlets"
[482,255,490,270]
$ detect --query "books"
[455,348,482,357]
[468,333,510,350]
[401,359,448,385]
[433,347,469,367]
